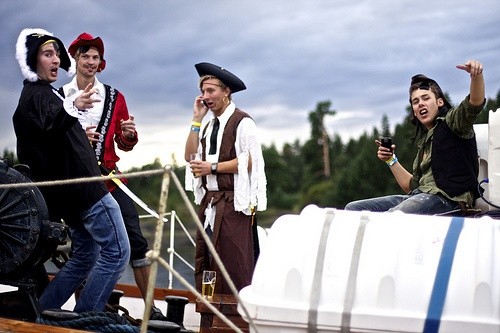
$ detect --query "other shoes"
[148,306,168,321]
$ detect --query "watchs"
[211,161,218,174]
[71,106,87,117]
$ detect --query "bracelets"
[384,155,400,167]
[192,121,200,131]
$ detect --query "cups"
[189,154,201,176]
[202,271,216,301]
[82,121,92,146]
[378,138,393,162]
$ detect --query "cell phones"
[202,100,209,108]
[380,137,393,156]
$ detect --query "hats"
[16,28,77,84]
[69,32,106,73]
[194,62,247,93]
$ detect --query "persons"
[185,62,266,294]
[58,32,168,321]
[12,27,131,315]
[345,60,486,215]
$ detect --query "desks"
[196,292,249,333]
